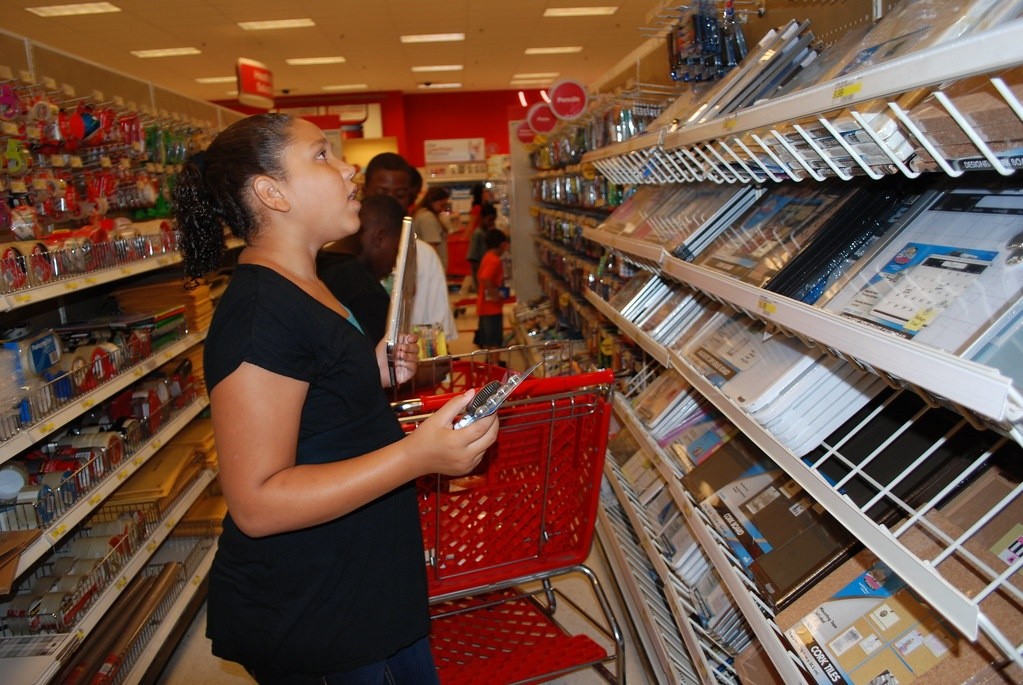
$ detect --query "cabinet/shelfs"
[419,153,512,288]
[509,0,1023,685]
[0,27,255,685]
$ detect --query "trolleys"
[392,338,627,684]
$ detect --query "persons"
[173,112,508,685]
